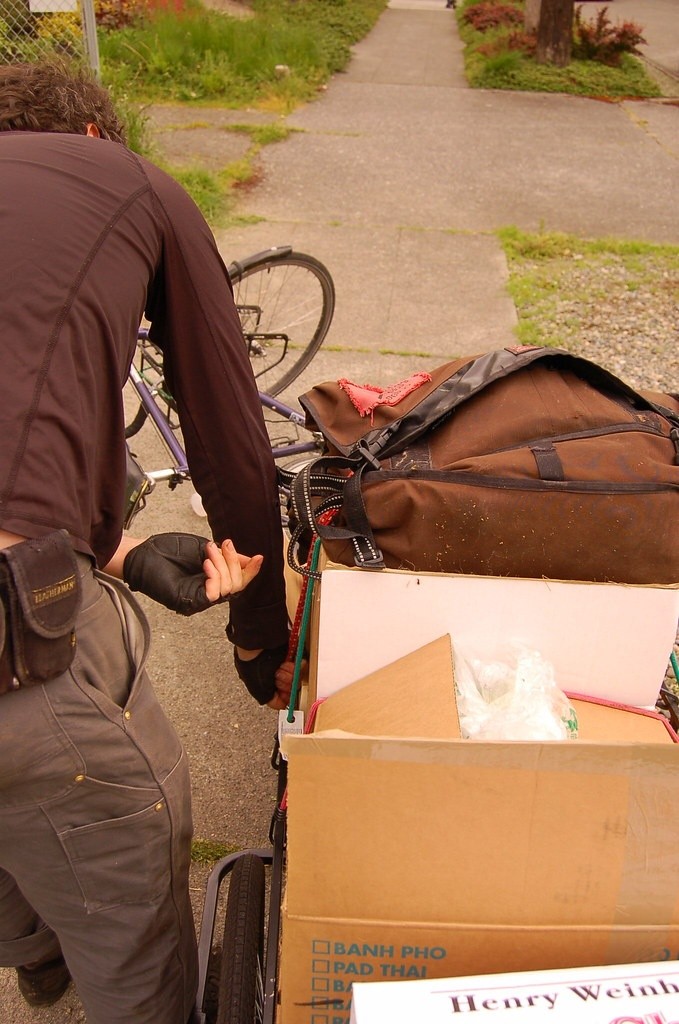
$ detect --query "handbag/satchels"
[287,341,679,585]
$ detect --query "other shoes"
[16,958,71,1008]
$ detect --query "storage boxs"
[276,538,679,1024]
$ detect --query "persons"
[0,58,308,1024]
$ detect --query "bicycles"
[124,248,331,533]
